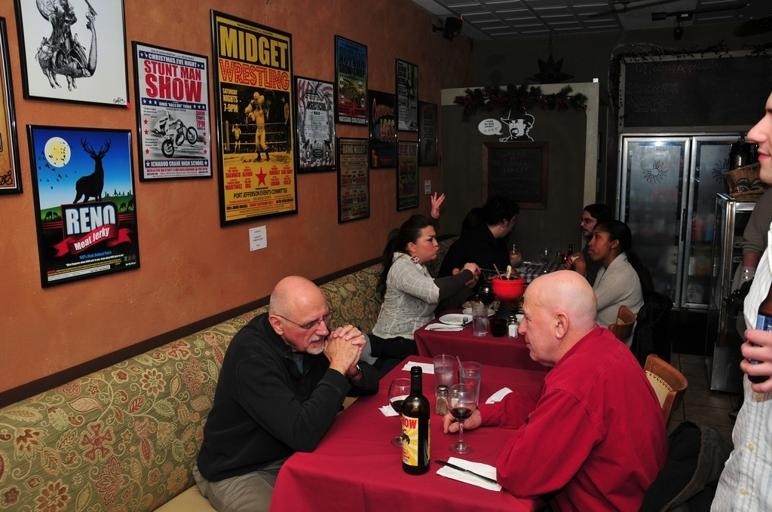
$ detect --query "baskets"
[720,163,765,201]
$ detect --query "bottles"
[745,287,772,383]
[400,365,433,475]
[508,315,519,338]
[434,383,450,416]
[567,243,575,256]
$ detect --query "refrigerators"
[616,131,745,315]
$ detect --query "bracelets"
[740,275,754,281]
[741,269,754,274]
[347,364,361,382]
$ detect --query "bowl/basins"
[492,276,524,301]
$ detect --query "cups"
[433,353,485,405]
[509,243,519,256]
[471,305,489,337]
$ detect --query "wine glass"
[444,381,477,457]
[385,378,410,447]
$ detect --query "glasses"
[273,312,333,329]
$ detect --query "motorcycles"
[162,118,198,158]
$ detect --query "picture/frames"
[27,124,141,288]
[481,141,547,211]
[132,41,213,182]
[0,15,24,195]
[14,0,131,108]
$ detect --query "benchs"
[0,234,464,511]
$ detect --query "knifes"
[434,459,498,486]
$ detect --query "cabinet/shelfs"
[702,190,758,395]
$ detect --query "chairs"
[630,292,673,359]
[607,306,633,343]
[643,352,688,431]
[645,421,719,511]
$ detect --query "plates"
[439,313,473,326]
[462,308,495,318]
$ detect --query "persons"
[709,93,771,512]
[461,206,523,266]
[191,277,378,512]
[739,185,771,289]
[573,204,652,304]
[437,196,519,311]
[557,220,645,349]
[369,192,480,371]
[245,92,270,161]
[232,123,241,153]
[441,270,669,511]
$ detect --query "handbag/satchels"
[669,420,701,463]
[467,281,517,320]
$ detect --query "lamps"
[672,14,685,40]
